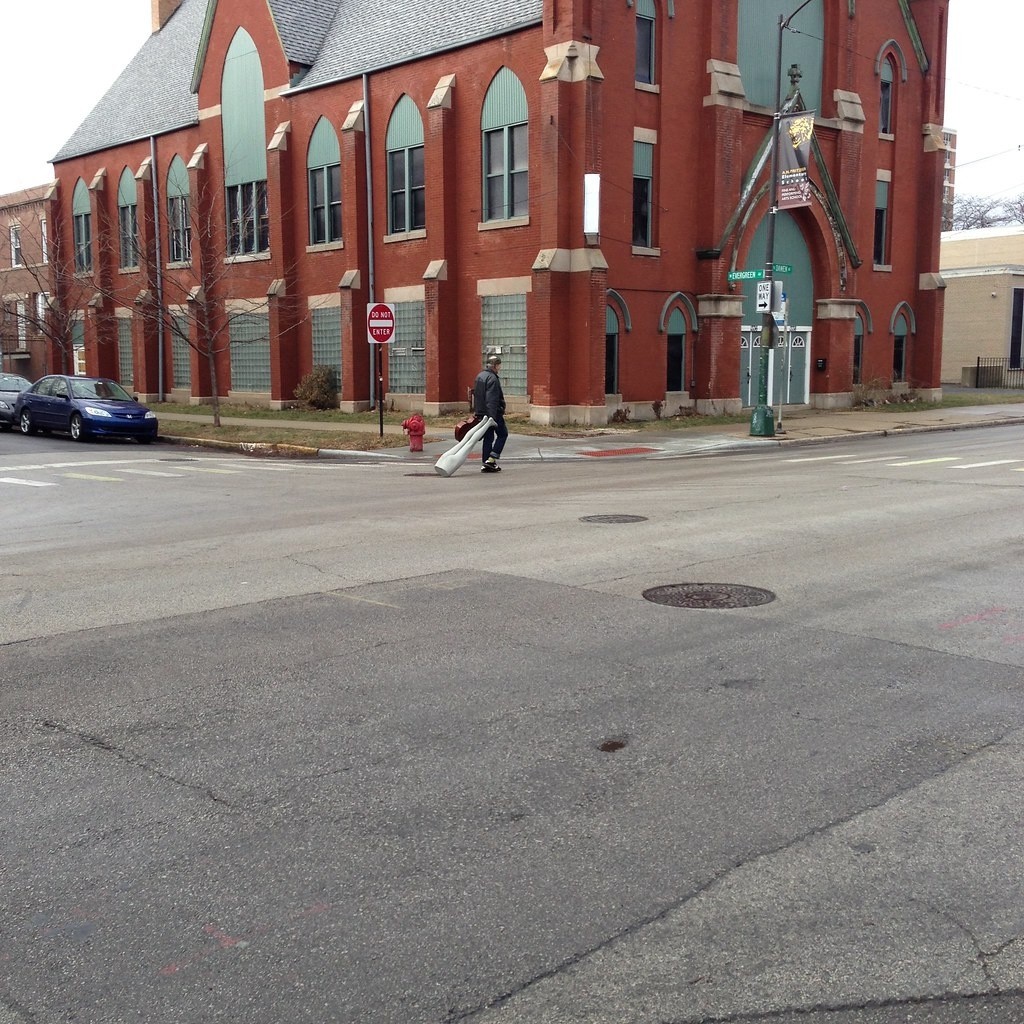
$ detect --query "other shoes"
[481,465,498,473]
[484,461,502,471]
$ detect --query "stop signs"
[368,303,395,343]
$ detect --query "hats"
[487,356,502,364]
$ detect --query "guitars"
[455,415,486,442]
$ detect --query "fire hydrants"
[401,413,425,452]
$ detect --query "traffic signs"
[756,281,772,311]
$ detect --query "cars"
[0,372,33,433]
[14,374,159,446]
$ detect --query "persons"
[475,355,508,472]
